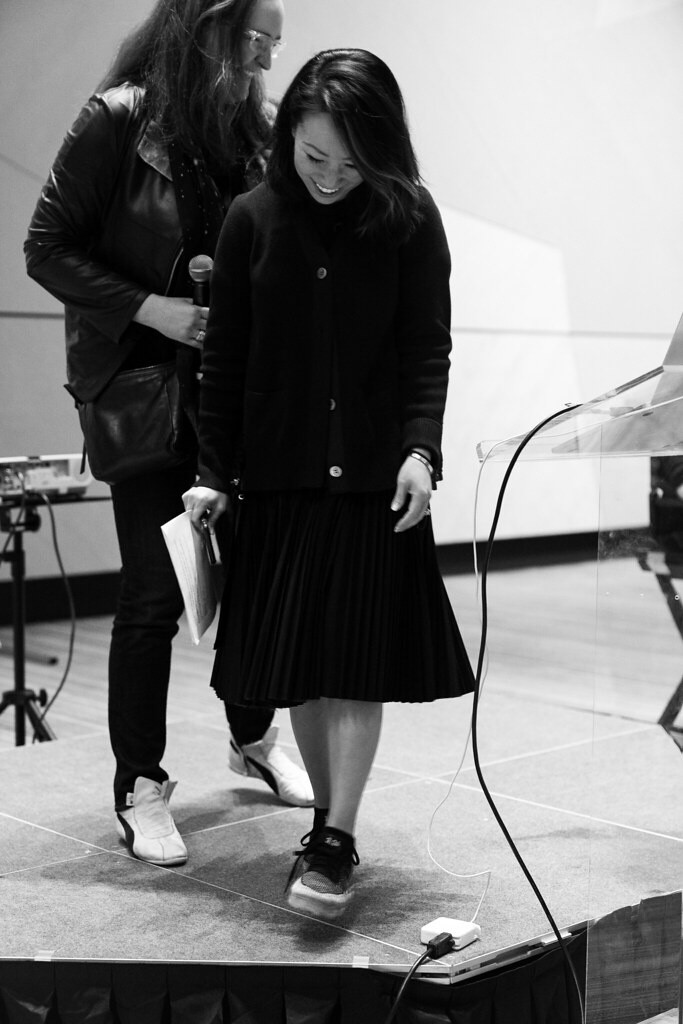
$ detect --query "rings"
[194,331,205,341]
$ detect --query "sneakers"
[116,775,188,868]
[228,725,315,807]
[284,807,360,920]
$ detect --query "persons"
[180,45,476,919]
[22,2,283,867]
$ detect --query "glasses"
[244,26,286,58]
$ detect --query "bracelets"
[406,450,433,477]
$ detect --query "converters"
[420,916,482,951]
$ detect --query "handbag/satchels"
[77,363,181,487]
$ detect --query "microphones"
[188,255,213,307]
